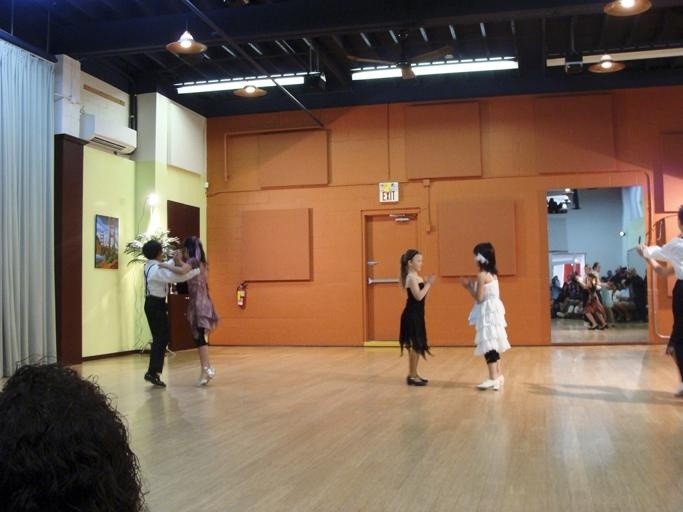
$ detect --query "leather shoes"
[407,376,425,386]
[498,375,505,385]
[144,372,166,387]
[421,378,428,383]
[476,378,500,390]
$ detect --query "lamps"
[587,12,626,73]
[349,56,519,81]
[603,0,652,17]
[546,44,683,67]
[232,85,268,97]
[165,4,207,54]
[564,14,583,72]
[173,71,322,94]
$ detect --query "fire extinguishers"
[237,281,246,306]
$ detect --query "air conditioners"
[80,112,138,155]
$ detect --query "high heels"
[600,323,608,330]
[588,324,599,330]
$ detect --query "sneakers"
[199,367,216,385]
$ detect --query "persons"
[551,261,648,330]
[141,241,205,388]
[457,242,512,391]
[0,354,147,512]
[398,249,437,386]
[634,202,683,398]
[158,234,219,388]
